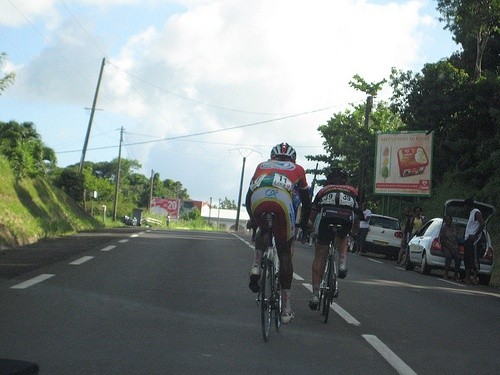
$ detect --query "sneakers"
[309,296,319,310]
[249,265,261,293]
[338,265,347,279]
[281,311,295,323]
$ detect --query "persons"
[349,203,372,257]
[306,169,365,311]
[439,214,461,282]
[245,141,312,324]
[166,213,171,227]
[460,199,485,284]
[396,205,426,270]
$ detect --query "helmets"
[271,142,296,161]
[327,168,348,179]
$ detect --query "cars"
[348,214,405,259]
[405,199,497,285]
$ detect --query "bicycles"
[308,232,355,323]
[247,222,306,342]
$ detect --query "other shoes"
[397,262,400,264]
[443,276,448,280]
[452,278,461,282]
[354,252,361,255]
[463,280,478,286]
